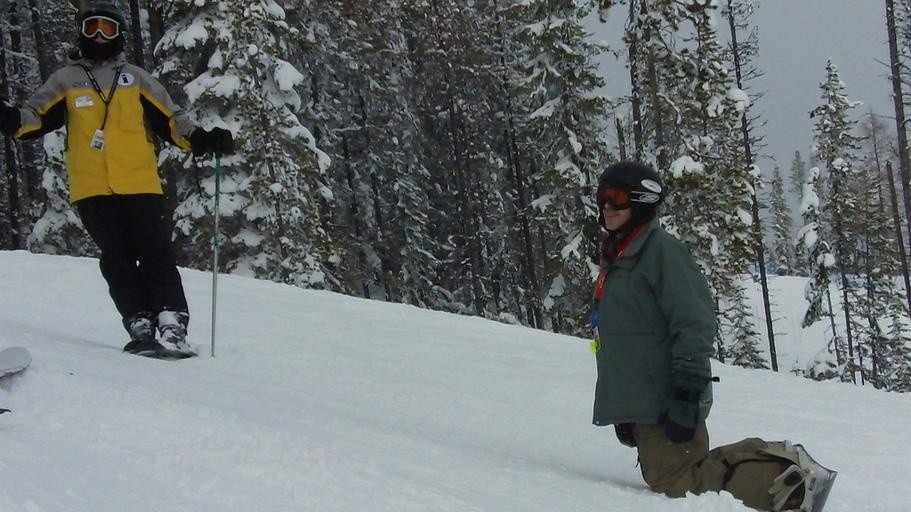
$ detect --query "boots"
[122,304,198,359]
[765,440,837,512]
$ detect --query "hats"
[77,0,129,32]
[597,162,665,211]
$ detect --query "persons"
[0,0,233,360]
[591,161,837,512]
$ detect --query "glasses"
[596,185,632,210]
[81,16,120,39]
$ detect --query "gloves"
[658,387,701,442]
[614,423,637,447]
[189,126,236,157]
[0,99,22,137]
